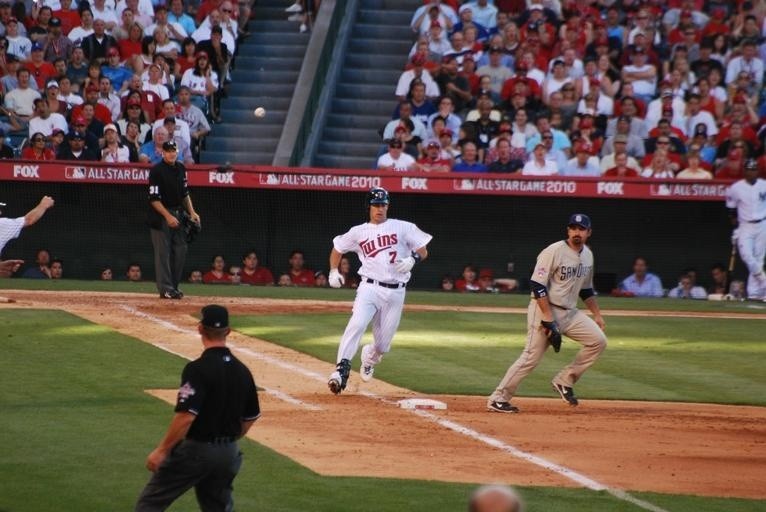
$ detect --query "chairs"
[0,93,211,159]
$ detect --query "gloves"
[396,254,419,275]
[540,319,562,353]
[326,267,346,288]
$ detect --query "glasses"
[222,8,232,14]
[229,272,241,276]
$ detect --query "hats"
[744,159,758,170]
[0,0,224,141]
[367,186,392,205]
[478,268,494,278]
[162,140,177,152]
[188,303,229,328]
[564,212,592,231]
[389,3,747,157]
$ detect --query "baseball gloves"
[541,320,561,352]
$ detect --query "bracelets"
[411,252,421,261]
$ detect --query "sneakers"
[486,400,520,414]
[551,378,579,406]
[326,370,343,396]
[358,342,378,383]
[284,2,309,33]
[159,288,184,300]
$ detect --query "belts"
[736,215,766,224]
[546,302,569,312]
[208,435,239,444]
[360,273,408,290]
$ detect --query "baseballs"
[254,106,266,116]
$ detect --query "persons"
[485,211,607,412]
[469,484,524,511]
[134,304,263,512]
[326,186,434,394]
